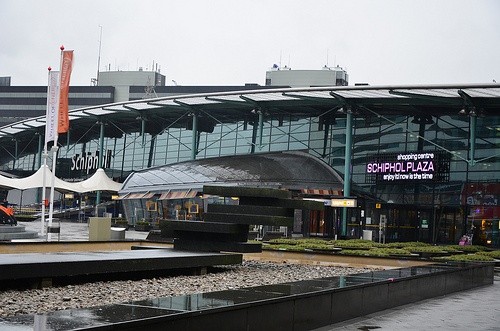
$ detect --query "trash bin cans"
[362,229,376,242]
[111,225,127,240]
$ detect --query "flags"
[44,70,59,143]
[58,47,75,134]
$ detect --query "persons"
[3,197,9,224]
[467,222,484,247]
